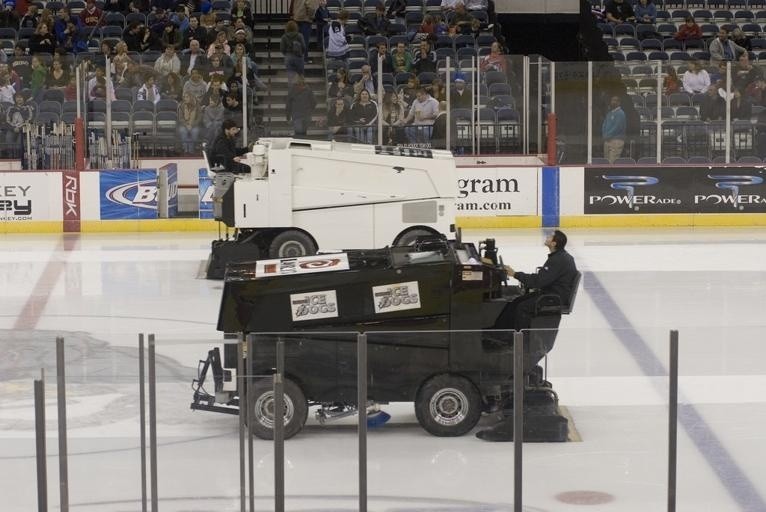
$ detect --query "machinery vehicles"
[188,233,585,445]
[193,136,460,278]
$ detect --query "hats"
[5,2,15,6]
[454,73,464,82]
[235,28,246,34]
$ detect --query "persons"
[482,230,576,349]
[601,94,627,164]
[606,0,635,28]
[662,14,765,136]
[634,1,657,25]
[2,0,510,156]
[210,119,251,227]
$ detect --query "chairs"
[535,271,581,315]
[202,140,235,202]
[0,0,766,165]
